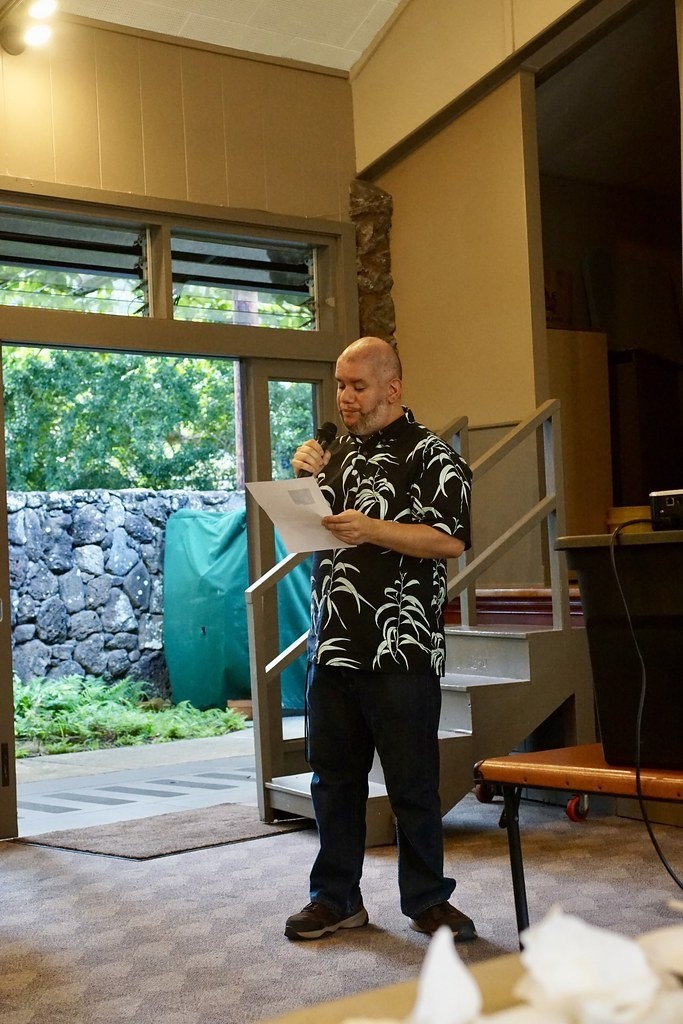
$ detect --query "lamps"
[0,0,58,57]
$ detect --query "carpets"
[7,802,316,863]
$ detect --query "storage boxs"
[552,531,683,773]
[606,505,653,533]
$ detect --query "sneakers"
[408,903,479,943]
[286,900,369,939]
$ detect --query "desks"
[468,741,683,951]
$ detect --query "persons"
[284,336,479,943]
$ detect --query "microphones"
[296,421,338,478]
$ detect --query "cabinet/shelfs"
[545,330,609,537]
